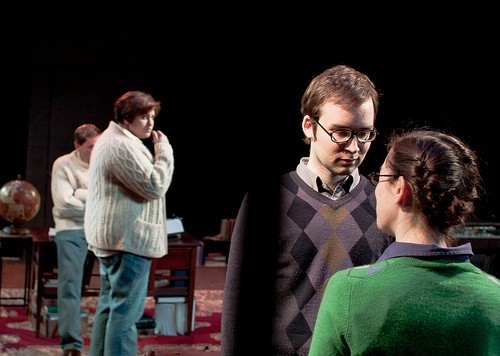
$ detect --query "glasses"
[368,172,399,186]
[313,118,379,144]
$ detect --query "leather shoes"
[62,350,82,356]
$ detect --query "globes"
[0,174,41,236]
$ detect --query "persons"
[83,92,174,356]
[221,65,396,356]
[306,130,500,356]
[51,124,103,356]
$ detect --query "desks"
[26,228,200,339]
[0,231,33,316]
